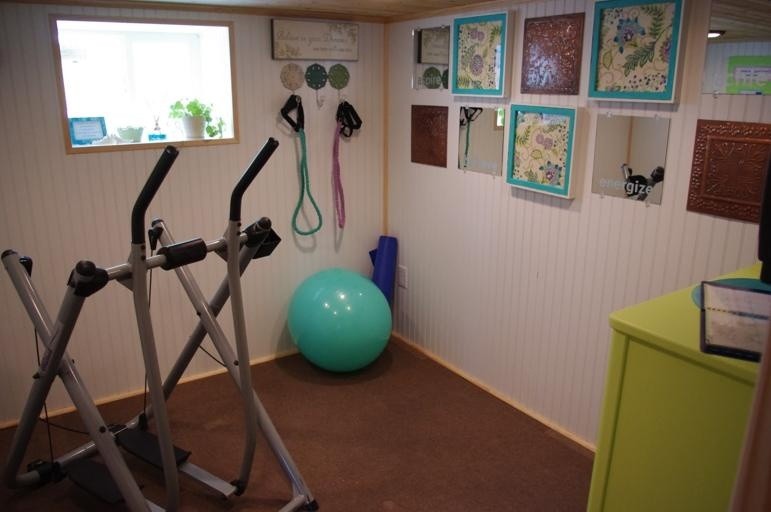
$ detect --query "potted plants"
[169,100,224,139]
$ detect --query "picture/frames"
[68,117,107,145]
[449,10,515,98]
[585,0,685,103]
[418,30,448,65]
[505,103,583,200]
[271,18,360,61]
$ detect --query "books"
[700,280,771,363]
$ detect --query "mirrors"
[591,113,670,205]
[698,1,771,95]
[458,105,504,176]
[409,24,450,93]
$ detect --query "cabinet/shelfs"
[588,262,762,512]
